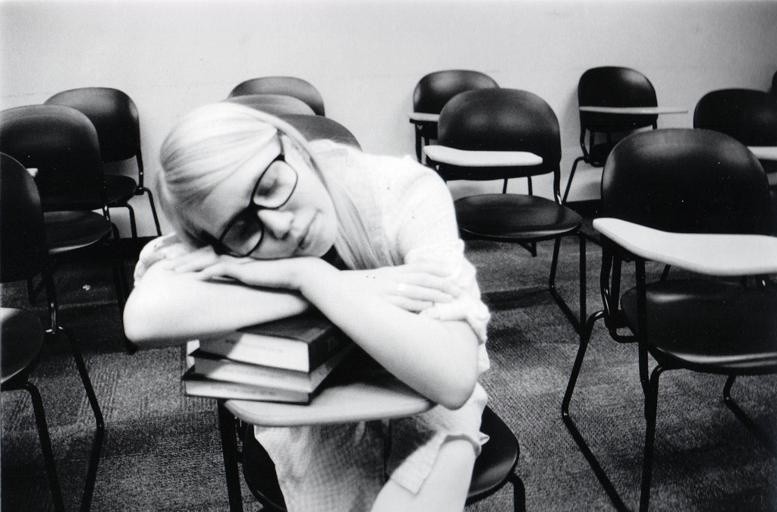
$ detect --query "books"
[196,309,347,374]
[180,364,328,405]
[187,348,342,393]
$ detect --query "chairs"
[186,331,528,510]
[558,66,688,234]
[0,105,134,357]
[556,130,776,510]
[421,86,587,333]
[410,70,541,260]
[0,153,103,511]
[221,65,777,190]
[27,86,161,308]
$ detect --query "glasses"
[211,131,300,260]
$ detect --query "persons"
[123,104,493,511]
